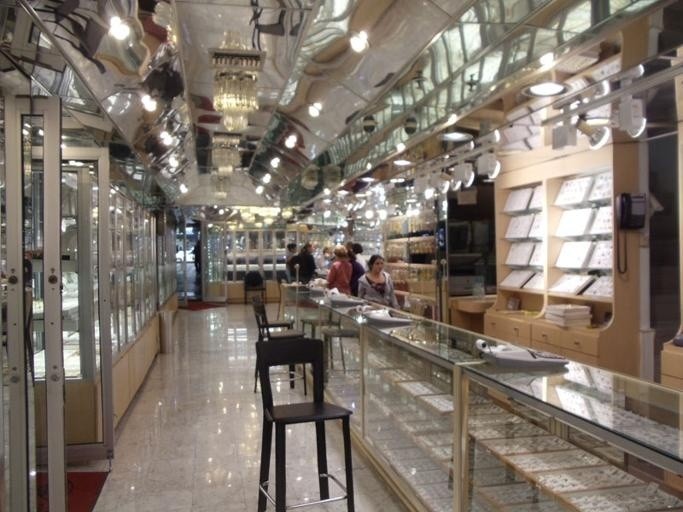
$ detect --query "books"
[544,303,593,329]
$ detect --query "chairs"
[255,338,353,512]
[243,271,267,303]
[253,300,295,388]
[322,326,358,373]
[301,310,342,344]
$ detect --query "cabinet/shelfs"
[395,293,437,319]
[222,230,286,282]
[495,168,613,330]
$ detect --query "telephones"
[617,192,647,234]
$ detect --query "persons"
[347,249,364,297]
[284,244,297,282]
[287,244,316,284]
[352,243,370,272]
[357,255,400,309]
[326,244,352,296]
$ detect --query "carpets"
[180,302,217,310]
[36,471,110,512]
